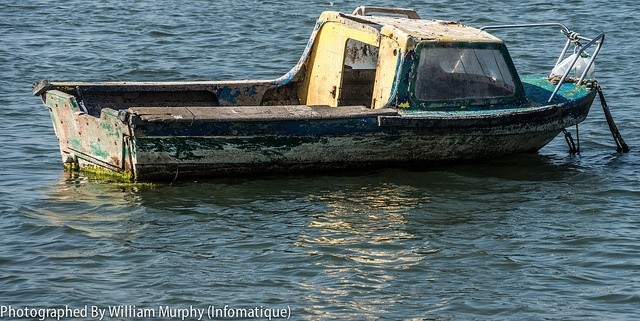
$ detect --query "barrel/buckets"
[550,52,595,78]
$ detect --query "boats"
[32,6,605,186]
